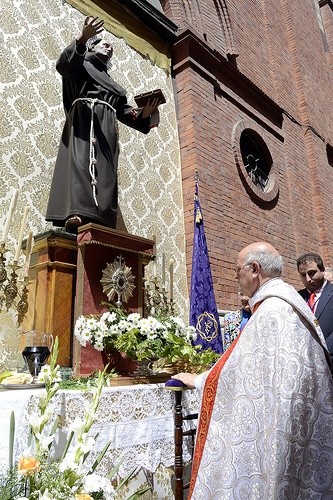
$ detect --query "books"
[134,89,167,107]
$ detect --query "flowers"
[74,301,221,376]
[0,335,152,500]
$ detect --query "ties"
[308,291,316,314]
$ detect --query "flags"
[189,182,223,353]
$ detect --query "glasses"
[232,263,252,273]
[237,291,244,296]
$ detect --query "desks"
[0,382,201,500]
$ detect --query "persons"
[170,241,333,500]
[224,288,251,353]
[296,253,333,371]
[46,15,162,233]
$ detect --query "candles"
[24,231,33,277]
[14,207,30,261]
[2,189,19,242]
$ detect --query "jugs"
[21,330,53,378]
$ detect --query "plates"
[0,382,45,389]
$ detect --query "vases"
[130,360,156,376]
